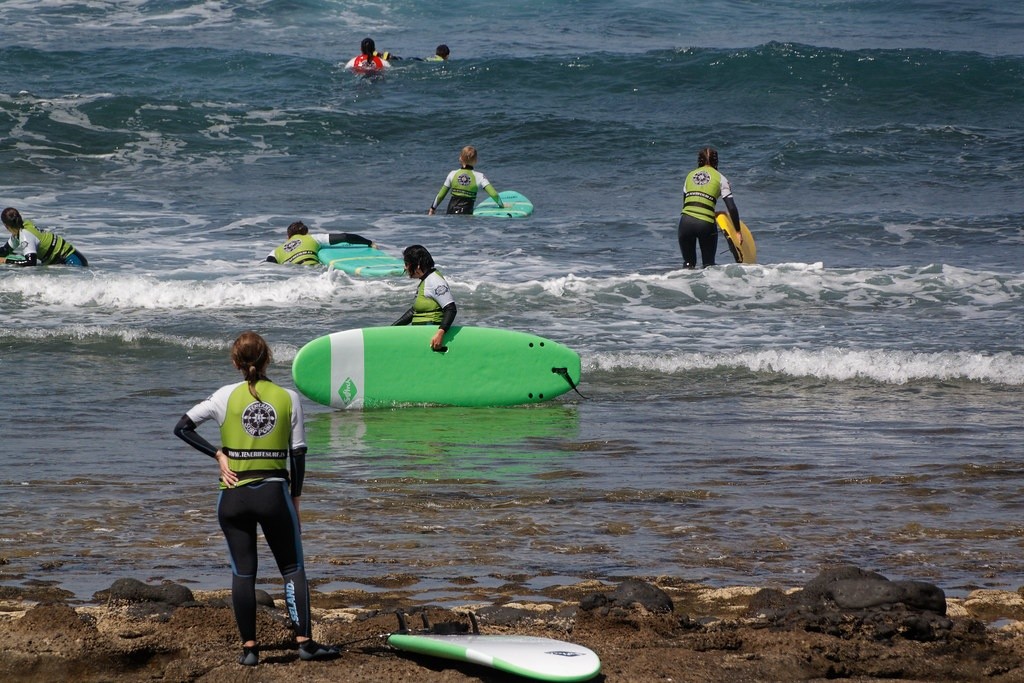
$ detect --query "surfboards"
[290,322,581,414]
[318,241,407,275]
[385,634,601,683]
[714,210,757,264]
[471,190,534,219]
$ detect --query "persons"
[390,245,457,350]
[424,44,450,62]
[677,148,743,269]
[0,208,88,267]
[266,220,379,268]
[345,38,391,69]
[429,146,504,215]
[174,331,340,665]
